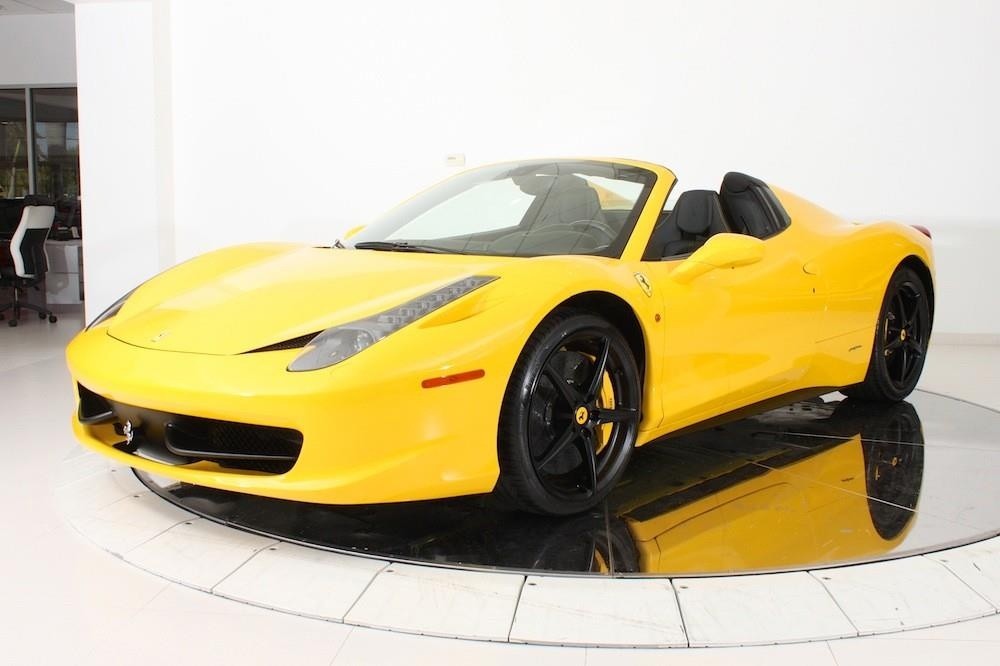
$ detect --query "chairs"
[647,188,734,258]
[0,195,58,326]
[518,175,612,249]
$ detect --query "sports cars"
[64,154,938,523]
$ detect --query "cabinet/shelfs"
[43,238,84,305]
